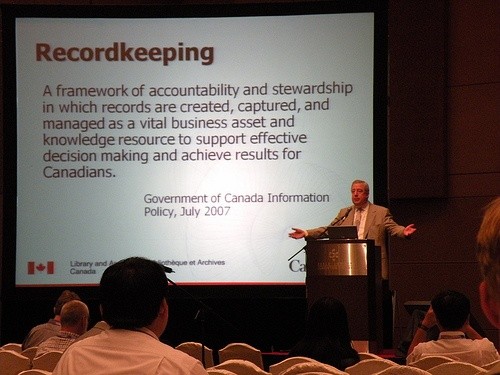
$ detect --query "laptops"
[327,225,359,239]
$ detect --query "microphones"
[160,265,173,273]
[342,206,352,220]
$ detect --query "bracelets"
[419,323,430,332]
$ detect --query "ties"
[355,207,362,234]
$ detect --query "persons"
[21,256,209,375]
[290,180,416,280]
[459,198,500,336]
[406,292,500,366]
[287,298,360,371]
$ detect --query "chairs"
[0,343,500,375]
